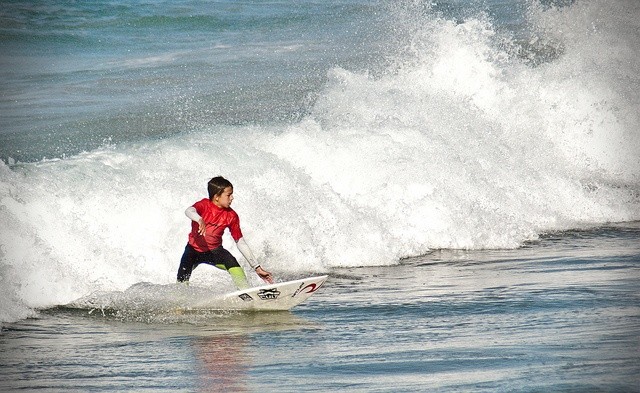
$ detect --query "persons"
[177,177,274,292]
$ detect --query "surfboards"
[192,274,329,311]
